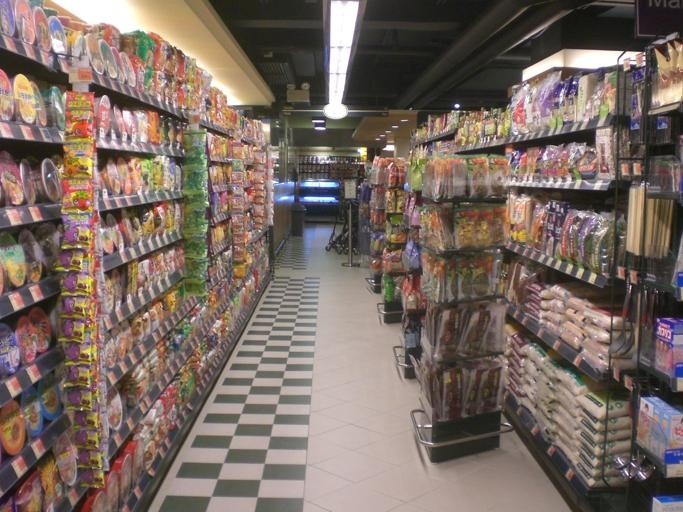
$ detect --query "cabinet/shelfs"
[0,0,272,512]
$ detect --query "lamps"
[322,0,368,119]
[285,83,312,104]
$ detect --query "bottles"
[300,156,359,179]
